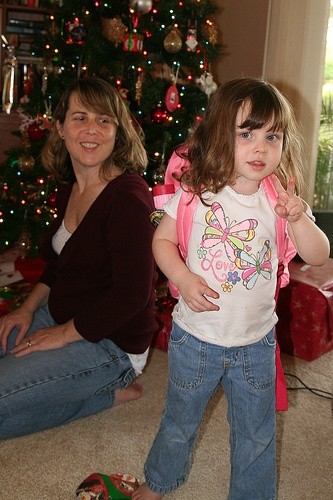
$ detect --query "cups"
[153,183,175,197]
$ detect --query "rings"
[26,340,31,346]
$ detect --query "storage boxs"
[276,259,333,361]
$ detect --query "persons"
[133,78,329,500]
[0,77,155,437]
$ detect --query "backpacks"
[153,141,297,299]
